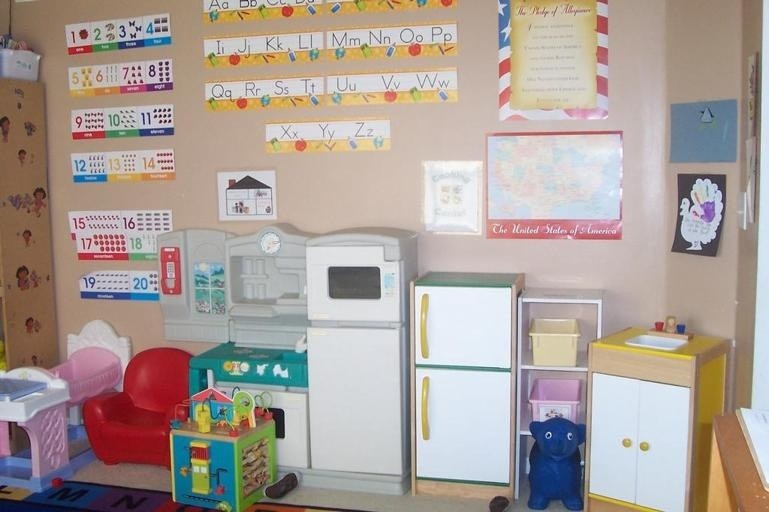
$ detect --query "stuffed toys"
[526,418,586,511]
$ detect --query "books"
[0,379,47,401]
